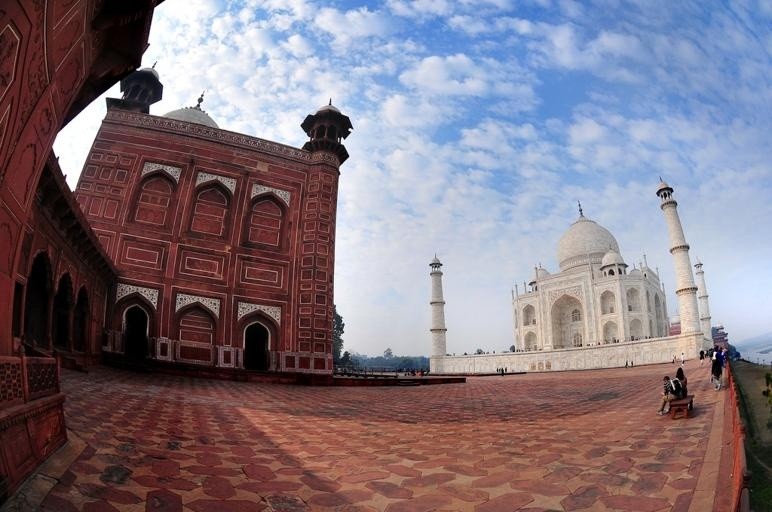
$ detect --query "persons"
[496,367,508,376]
[623,345,728,392]
[675,366,688,400]
[336,367,430,377]
[655,375,682,416]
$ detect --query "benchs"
[669,394,694,419]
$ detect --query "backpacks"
[670,378,682,395]
